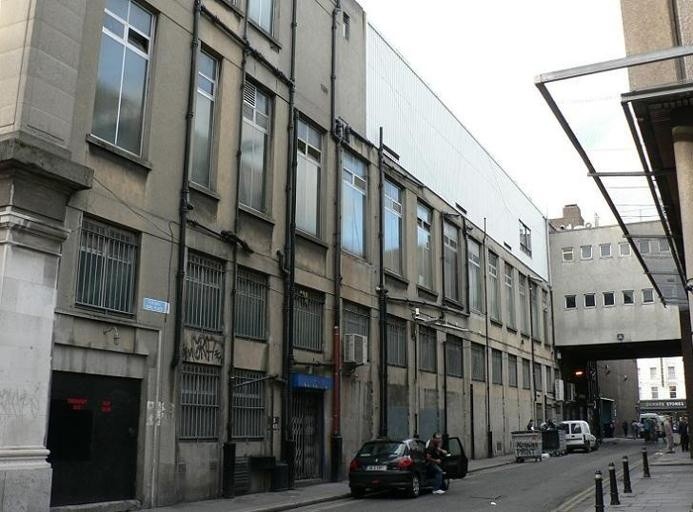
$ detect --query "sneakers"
[432,489,447,496]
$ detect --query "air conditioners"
[344,336,367,373]
[551,378,564,403]
[565,382,575,403]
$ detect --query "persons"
[622,415,689,453]
[426,431,450,494]
[527,419,535,429]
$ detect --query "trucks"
[660,415,666,432]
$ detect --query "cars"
[671,423,678,432]
[349,435,468,496]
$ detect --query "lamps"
[102,325,121,347]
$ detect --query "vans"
[637,413,664,438]
[559,421,596,451]
